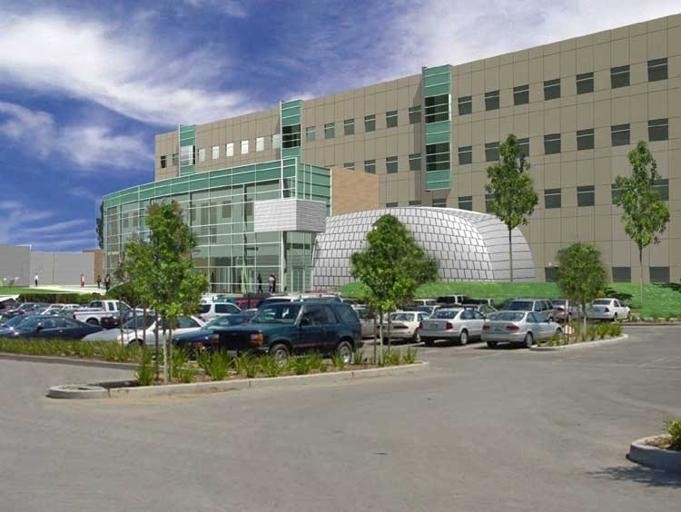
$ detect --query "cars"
[499,298,554,323]
[79,312,206,350]
[551,299,583,324]
[419,307,491,347]
[395,293,499,315]
[587,297,632,323]
[172,315,253,360]
[374,311,436,345]
[481,310,564,348]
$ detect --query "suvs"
[207,300,365,373]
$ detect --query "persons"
[80,273,85,287]
[271,273,276,292]
[104,274,111,291]
[96,275,102,288]
[34,273,38,286]
[268,273,275,292]
[256,273,263,294]
